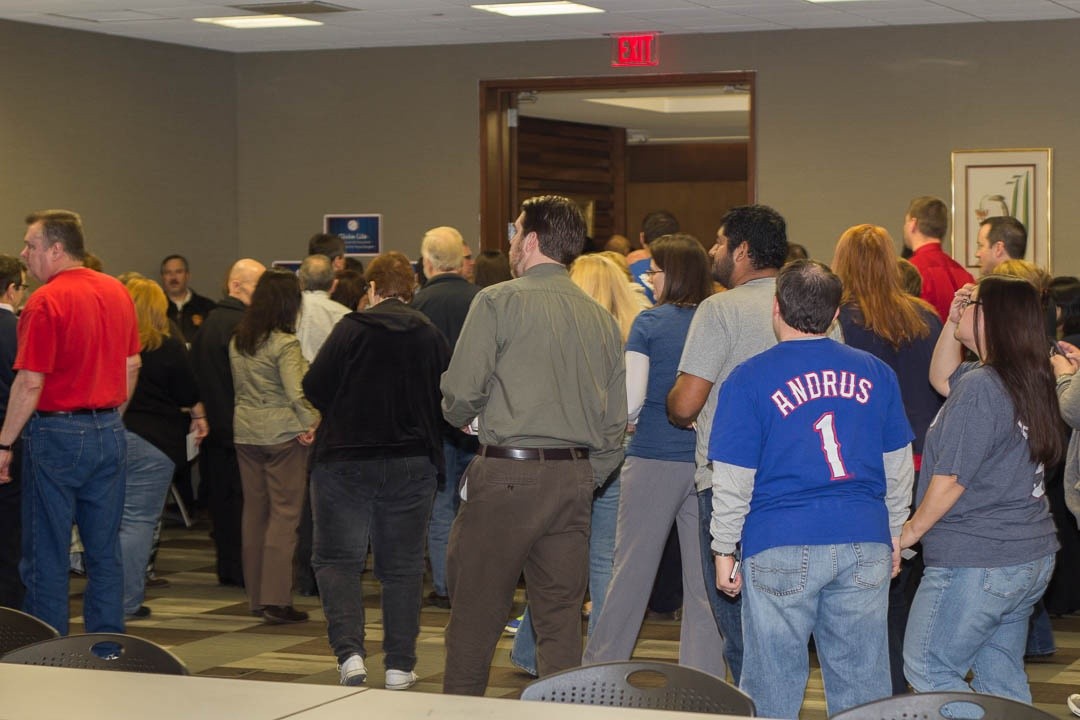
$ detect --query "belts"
[477,444,590,459]
[34,408,114,416]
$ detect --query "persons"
[0,195,1080,720]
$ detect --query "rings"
[731,591,733,594]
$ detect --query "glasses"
[16,284,28,292]
[968,297,983,307]
[645,269,664,280]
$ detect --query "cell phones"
[730,561,741,583]
[1049,336,1072,365]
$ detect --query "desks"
[0,663,770,720]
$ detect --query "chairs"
[519,659,758,718]
[830,692,1060,720]
[0,607,191,676]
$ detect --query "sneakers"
[385,667,416,690]
[336,655,368,686]
[253,607,310,624]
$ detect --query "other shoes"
[122,606,150,618]
[582,604,590,620]
[505,619,522,632]
[421,591,450,609]
[146,577,168,589]
[1067,693,1080,716]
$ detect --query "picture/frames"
[951,146,1053,283]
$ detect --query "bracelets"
[0,444,13,450]
[712,550,735,556]
[192,415,206,419]
